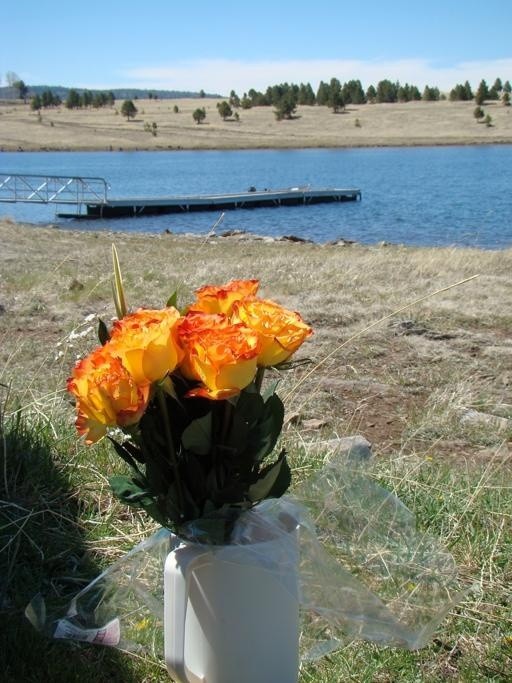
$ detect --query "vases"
[164,506,300,683]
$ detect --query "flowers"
[67,243,316,549]
